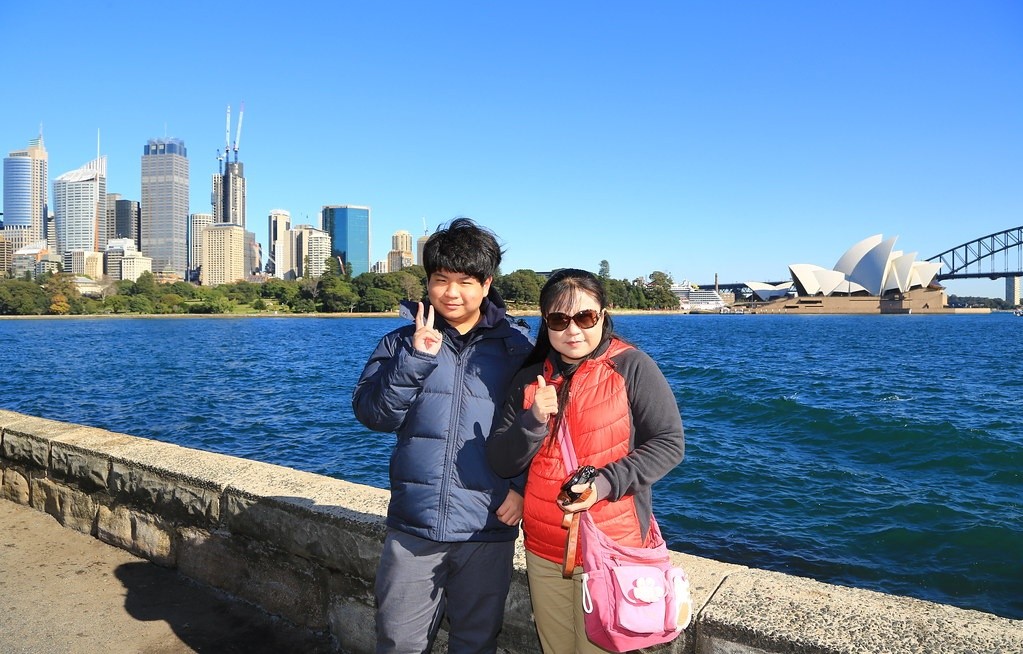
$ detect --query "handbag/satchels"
[580,512,692,654]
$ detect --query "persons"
[352,218,534,654]
[488,269,684,654]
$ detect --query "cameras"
[556,465,597,505]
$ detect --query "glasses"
[544,308,602,331]
